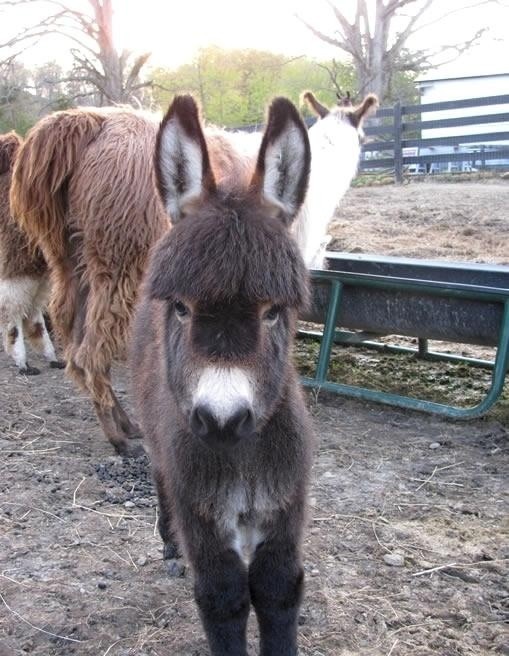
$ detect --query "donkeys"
[118,92,322,656]
[1,87,379,460]
